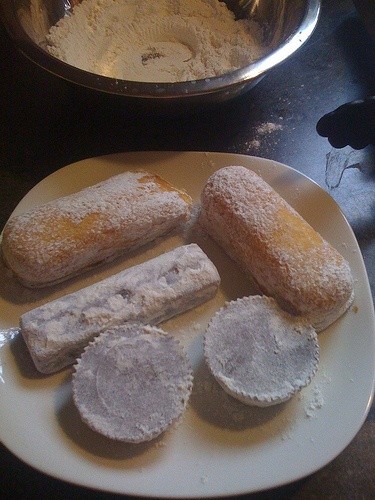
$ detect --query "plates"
[0,152,375,498]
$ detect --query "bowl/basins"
[0,0,322,100]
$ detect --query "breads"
[203,294,320,408]
[19,244,221,374]
[198,165,358,334]
[4,168,194,290]
[72,323,195,443]
